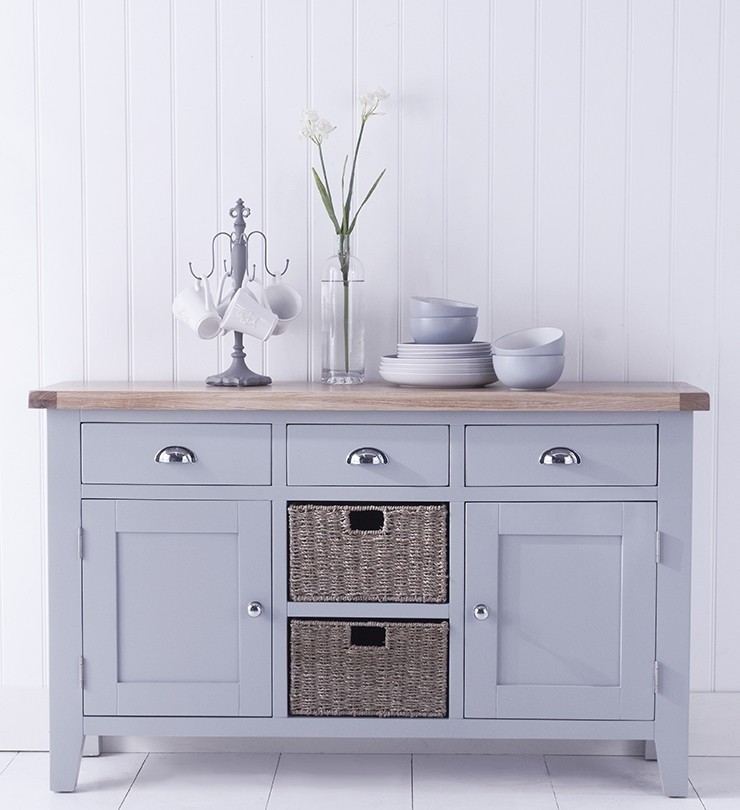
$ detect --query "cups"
[172,271,304,342]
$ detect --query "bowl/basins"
[407,294,480,344]
[492,326,566,393]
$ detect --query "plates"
[378,341,499,390]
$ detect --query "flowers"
[297,86,390,374]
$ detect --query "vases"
[318,234,367,385]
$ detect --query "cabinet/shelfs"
[31,381,709,795]
